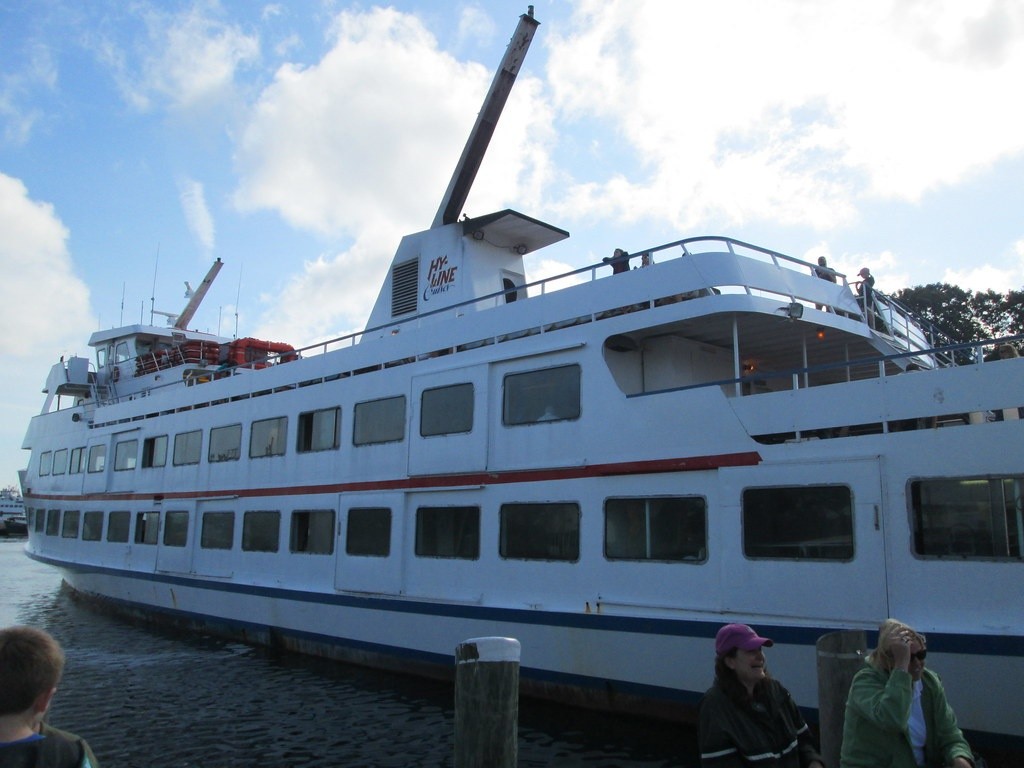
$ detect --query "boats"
[0,491,26,530]
[17,6,1024,768]
[4,516,27,531]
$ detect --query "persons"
[700,621,827,768]
[838,621,974,768]
[987,344,1024,422]
[812,256,837,312]
[603,249,699,317]
[855,268,874,324]
[0,624,98,768]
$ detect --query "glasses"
[910,649,927,662]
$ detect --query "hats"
[715,624,773,655]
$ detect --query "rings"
[901,637,908,642]
